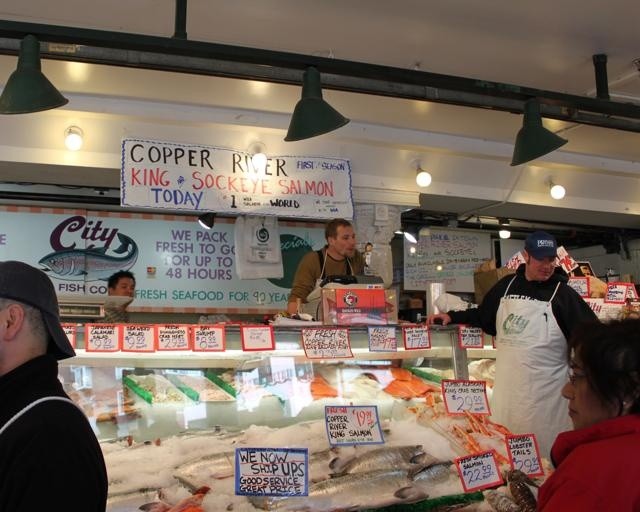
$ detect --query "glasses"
[567,370,587,384]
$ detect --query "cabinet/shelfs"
[58,326,507,512]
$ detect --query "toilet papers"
[425,282,446,317]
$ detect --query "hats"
[1,261,76,361]
[524,231,557,258]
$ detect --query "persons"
[92,271,135,323]
[580,266,590,276]
[426,231,601,470]
[288,219,369,314]
[536,317,640,512]
[0,261,108,512]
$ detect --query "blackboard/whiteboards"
[403,226,491,294]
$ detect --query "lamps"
[408,158,432,186]
[63,126,83,153]
[197,213,214,229]
[545,178,566,200]
[403,223,423,244]
[247,141,267,171]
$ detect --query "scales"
[56,300,106,323]
